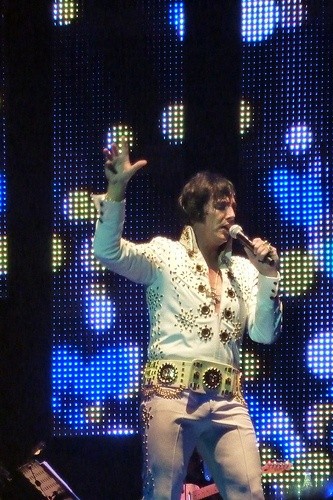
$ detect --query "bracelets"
[105,192,125,203]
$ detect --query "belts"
[141,358,242,398]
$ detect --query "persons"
[90,135,282,500]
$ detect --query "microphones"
[229,224,274,265]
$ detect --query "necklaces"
[204,264,220,305]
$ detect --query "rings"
[263,240,273,252]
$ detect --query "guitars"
[180,461,292,500]
